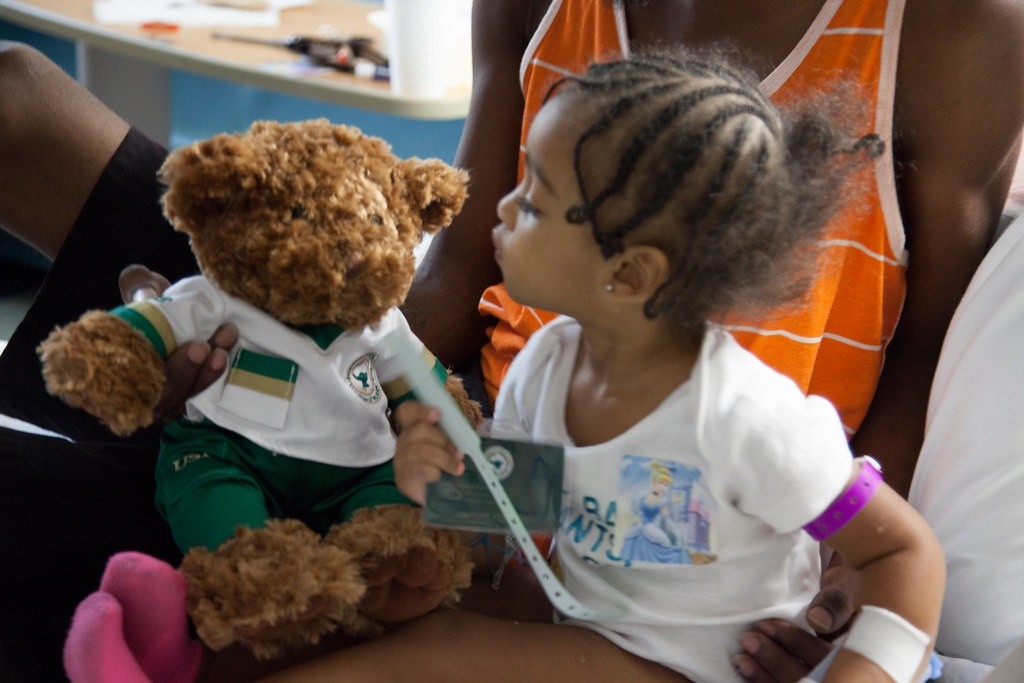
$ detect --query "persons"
[59,48,948,683]
[1,0,1024,683]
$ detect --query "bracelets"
[801,452,883,543]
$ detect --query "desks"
[0,0,474,151]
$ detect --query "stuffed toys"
[37,115,473,659]
